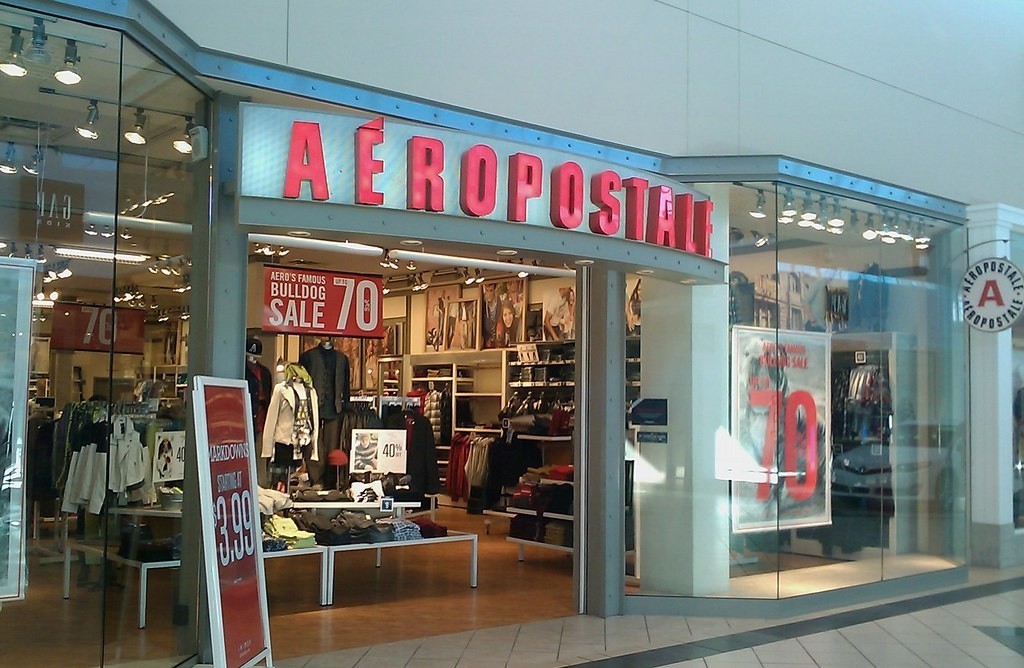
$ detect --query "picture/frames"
[363,323,396,392]
[443,297,477,351]
[476,277,529,348]
[425,282,462,352]
[299,335,328,355]
[332,338,361,391]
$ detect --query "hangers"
[454,431,500,446]
[71,400,143,428]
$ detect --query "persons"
[738,354,827,522]
[432,283,575,351]
[299,340,350,420]
[304,326,394,390]
[261,362,319,468]
[245,337,272,434]
[625,279,641,336]
[354,432,378,470]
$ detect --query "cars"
[831,420,965,511]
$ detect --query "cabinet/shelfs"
[410,349,476,492]
[503,345,578,439]
[455,391,502,432]
[504,478,637,562]
[61,502,477,627]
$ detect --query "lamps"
[411,273,429,291]
[798,189,844,235]
[76,99,100,138]
[464,268,485,284]
[0,141,20,175]
[54,37,81,85]
[174,118,196,154]
[125,105,148,145]
[23,149,43,175]
[381,281,391,295]
[777,185,797,225]
[85,224,192,323]
[863,203,931,250]
[0,26,28,78]
[749,190,765,219]
[8,241,47,263]
